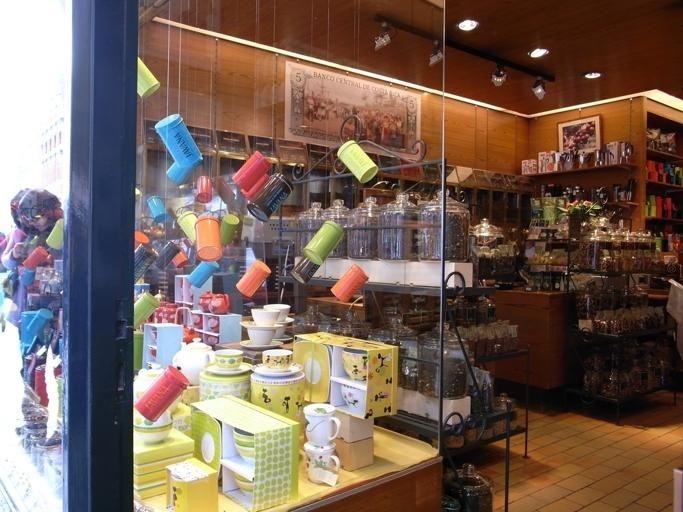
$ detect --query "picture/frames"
[555,114,603,155]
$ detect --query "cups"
[169,475,186,510]
[301,403,342,486]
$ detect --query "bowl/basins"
[226,430,256,500]
[135,409,173,446]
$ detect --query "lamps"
[371,17,556,102]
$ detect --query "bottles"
[442,462,496,511]
[131,56,479,421]
[438,392,519,448]
[576,215,683,397]
[8,218,65,352]
[475,219,507,253]
[645,164,673,220]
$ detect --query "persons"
[18,190,63,254]
[0,188,33,269]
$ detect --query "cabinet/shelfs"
[636,91,683,237]
